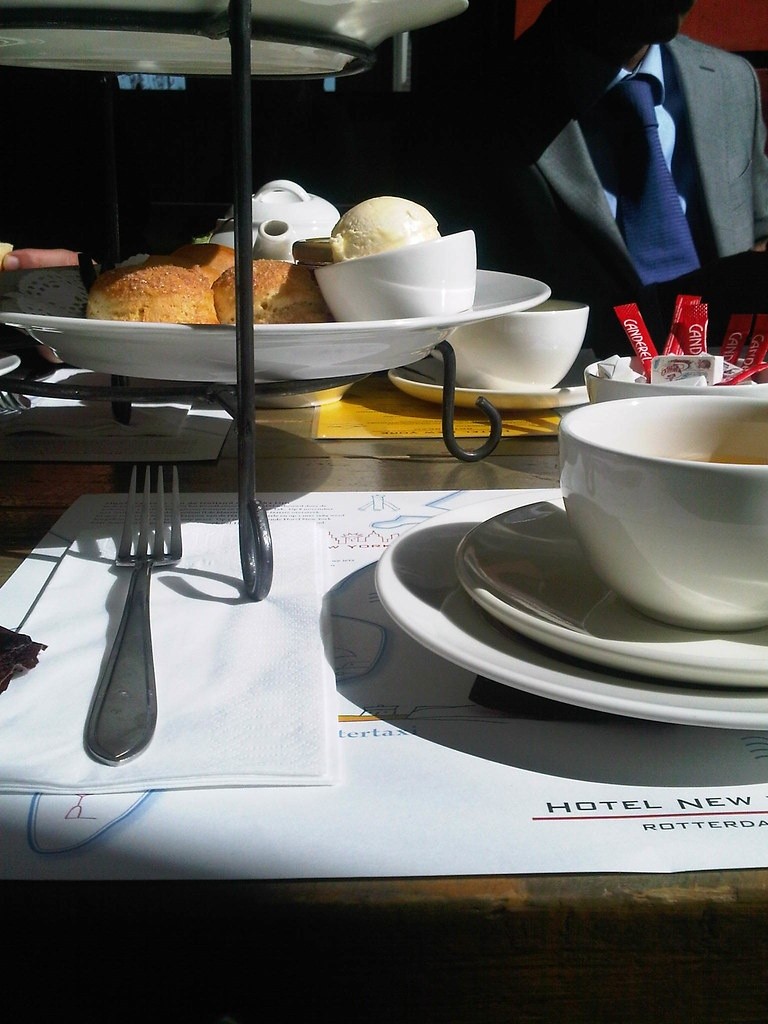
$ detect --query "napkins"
[0,521,346,794]
[4,368,194,437]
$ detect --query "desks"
[0,364,768,1024]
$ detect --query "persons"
[0,0,768,289]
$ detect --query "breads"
[86,242,341,325]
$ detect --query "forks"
[81,464,184,763]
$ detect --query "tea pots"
[211,177,342,262]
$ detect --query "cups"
[555,396,768,632]
[432,301,591,389]
[316,230,477,321]
[584,354,768,402]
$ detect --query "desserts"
[328,197,441,263]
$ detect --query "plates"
[374,502,768,732]
[386,365,598,410]
[454,499,768,691]
[254,372,372,409]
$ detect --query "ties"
[594,80,702,287]
